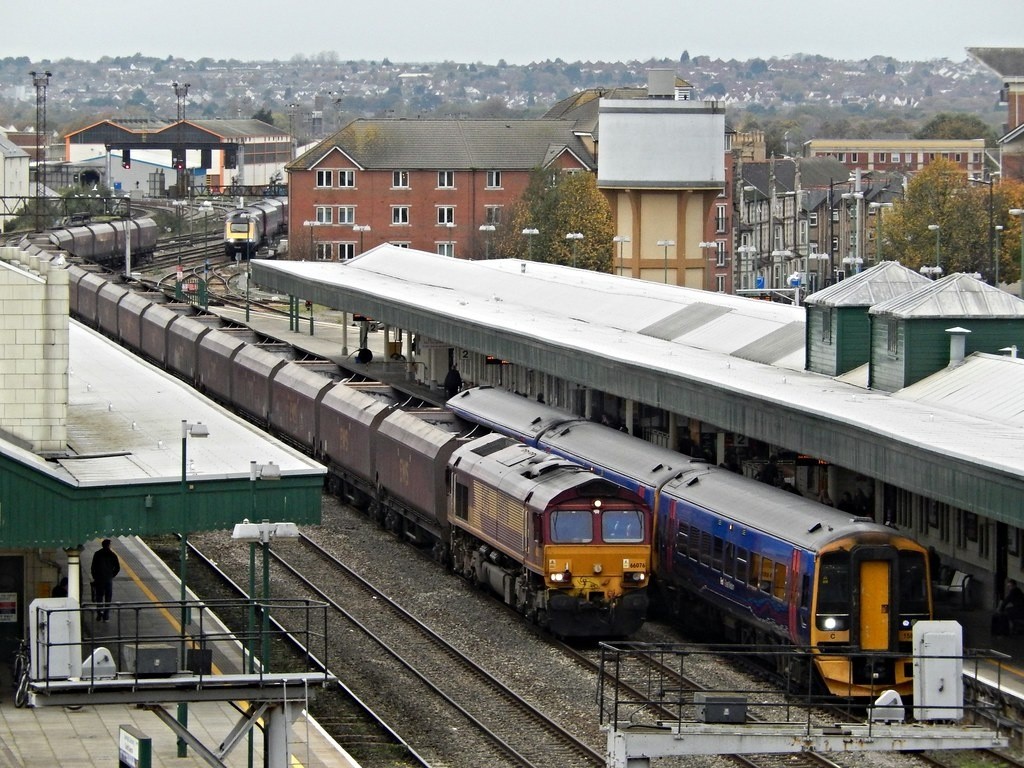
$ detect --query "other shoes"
[96,611,104,621]
[104,613,110,620]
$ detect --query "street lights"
[176,422,210,756]
[921,267,943,279]
[829,178,856,285]
[349,222,582,361]
[231,520,299,768]
[171,200,322,336]
[968,178,1024,301]
[928,225,941,279]
[743,185,757,289]
[658,240,864,307]
[868,201,892,263]
[784,190,811,289]
[840,192,862,275]
[248,461,281,768]
[612,237,632,277]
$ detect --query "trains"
[17,216,653,640]
[223,194,287,258]
[444,386,935,706]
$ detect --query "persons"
[514,390,801,496]
[52,577,69,598]
[90,538,121,622]
[444,365,462,400]
[837,488,896,525]
[925,545,940,580]
[996,579,1024,638]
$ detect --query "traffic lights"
[172,151,182,169]
[122,149,130,170]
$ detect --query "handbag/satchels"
[90,582,96,602]
